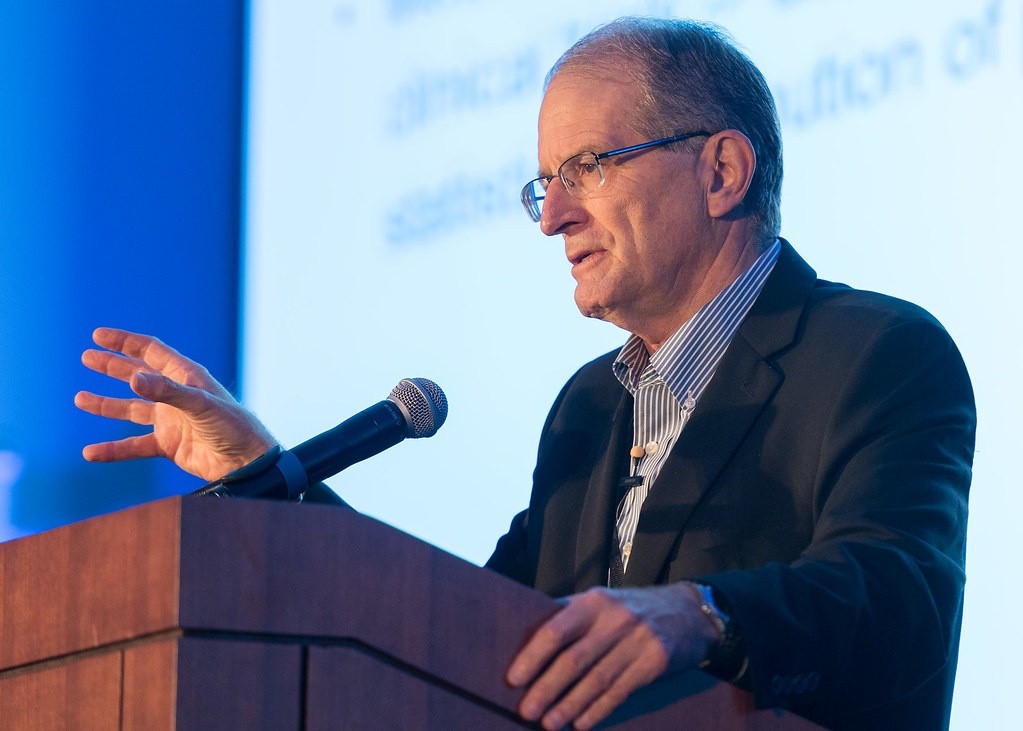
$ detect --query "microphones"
[188,376,449,499]
[631,445,645,464]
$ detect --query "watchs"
[686,578,735,654]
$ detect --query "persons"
[73,10,979,731]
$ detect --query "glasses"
[519,131,713,222]
